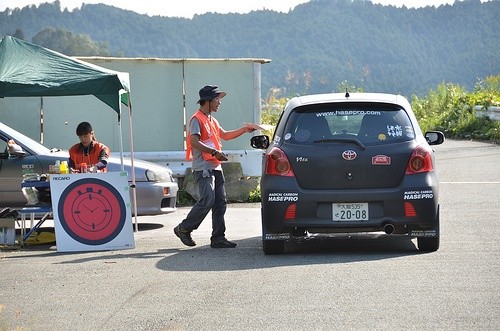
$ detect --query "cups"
[49,165,55,174]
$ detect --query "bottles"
[81,163,87,173]
[55,160,69,174]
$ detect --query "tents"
[0,35,138,232]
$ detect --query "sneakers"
[174,225,196,246]
[211,241,237,248]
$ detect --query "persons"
[68,121,109,173]
[174,85,256,248]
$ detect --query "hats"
[197,85,226,103]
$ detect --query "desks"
[18,180,55,245]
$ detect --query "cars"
[250,93,445,257]
[0,122,179,217]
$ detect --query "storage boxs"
[0,217,20,245]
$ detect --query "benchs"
[20,207,54,245]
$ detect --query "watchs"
[212,149,218,157]
